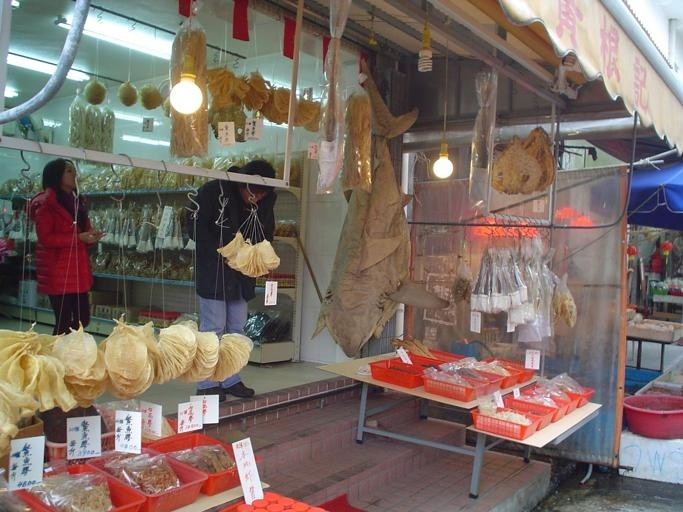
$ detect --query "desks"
[623,294,682,397]
[162,480,272,512]
[312,348,602,500]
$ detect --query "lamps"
[413,0,438,73]
[54,13,172,62]
[430,29,458,179]
[164,0,208,114]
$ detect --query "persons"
[185,158,278,403]
[29,157,107,336]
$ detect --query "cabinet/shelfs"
[1,150,308,370]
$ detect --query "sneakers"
[194,386,227,404]
[222,380,255,400]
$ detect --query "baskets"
[10,430,264,512]
[41,406,119,470]
[466,378,596,443]
[365,347,538,405]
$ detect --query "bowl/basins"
[623,394,683,439]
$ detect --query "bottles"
[67,88,115,153]
